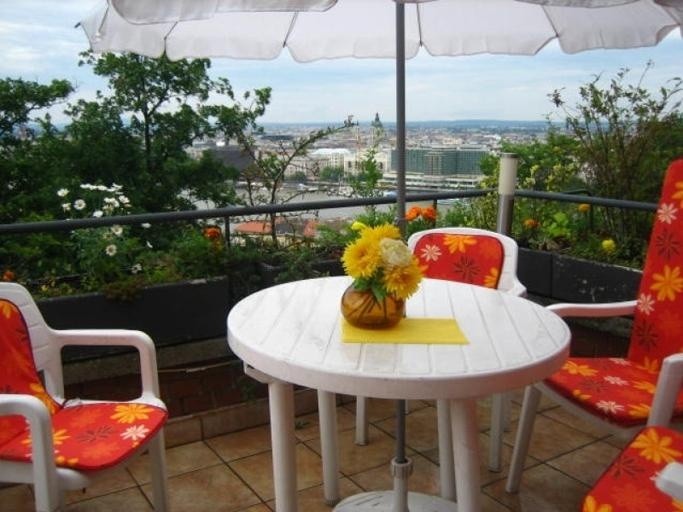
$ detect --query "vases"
[30,275,226,366]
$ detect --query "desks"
[226,275,572,512]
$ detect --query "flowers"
[0,174,428,329]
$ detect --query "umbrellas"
[70,2,683,465]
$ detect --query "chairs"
[0,280,169,512]
